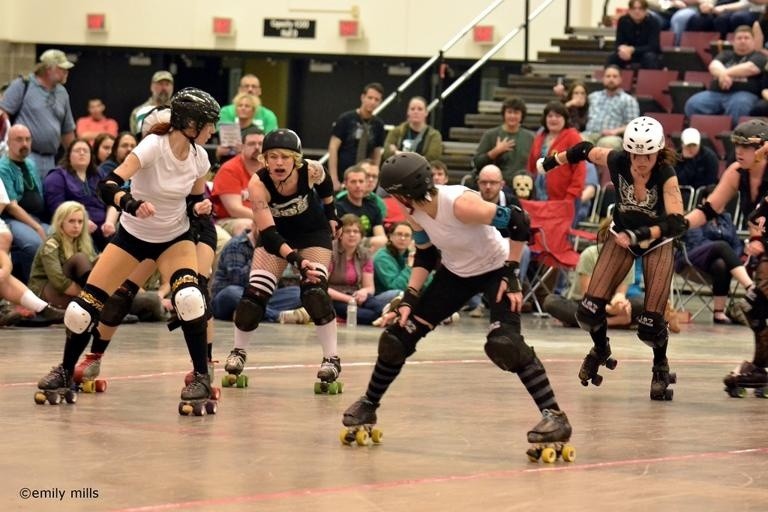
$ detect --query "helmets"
[169,87,220,124]
[377,151,434,201]
[731,119,767,145]
[623,116,666,155]
[262,128,303,155]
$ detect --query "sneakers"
[713,309,733,324]
[468,302,486,319]
[36,303,66,322]
[439,311,460,326]
[276,307,311,325]
[122,313,139,324]
[1,305,21,327]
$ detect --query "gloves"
[119,194,144,217]
[324,202,344,232]
[286,250,308,278]
[624,226,651,247]
[747,198,768,227]
[502,260,522,294]
[536,151,562,175]
[389,288,422,321]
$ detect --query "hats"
[680,127,701,147]
[41,49,75,70]
[151,70,175,86]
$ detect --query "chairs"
[591,70,632,95]
[662,30,721,72]
[635,68,679,113]
[577,183,601,221]
[667,70,713,108]
[691,113,731,185]
[572,180,613,256]
[656,31,676,64]
[642,112,684,163]
[518,196,597,318]
[738,115,768,126]
[675,186,742,332]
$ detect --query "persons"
[1,3,766,465]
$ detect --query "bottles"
[346,296,358,327]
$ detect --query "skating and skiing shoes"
[180,371,218,416]
[723,361,768,399]
[222,348,248,388]
[579,337,617,386]
[73,353,107,394]
[314,356,344,394]
[651,357,676,400]
[184,360,220,400]
[527,409,576,463]
[339,396,384,447]
[34,364,78,405]
